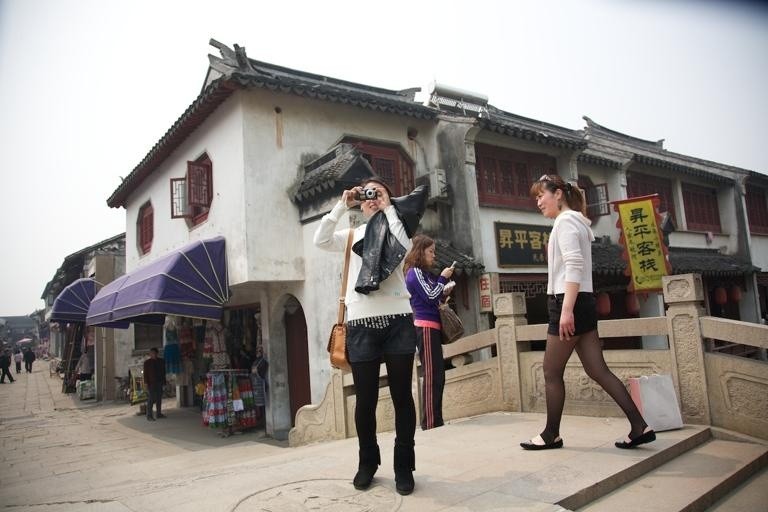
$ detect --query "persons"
[74,348,93,381]
[251,347,269,430]
[313,176,416,497]
[406,233,458,430]
[519,175,657,451]
[1,341,50,384]
[144,347,168,422]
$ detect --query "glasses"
[539,174,552,182]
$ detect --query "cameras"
[354,189,377,201]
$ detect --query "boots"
[353,445,380,489]
[394,446,415,495]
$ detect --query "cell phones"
[450,260,458,270]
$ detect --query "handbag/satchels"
[327,324,352,370]
[439,304,465,344]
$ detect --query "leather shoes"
[520,439,564,450]
[615,430,656,449]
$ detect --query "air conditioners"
[412,168,446,203]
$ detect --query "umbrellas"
[17,338,32,345]
